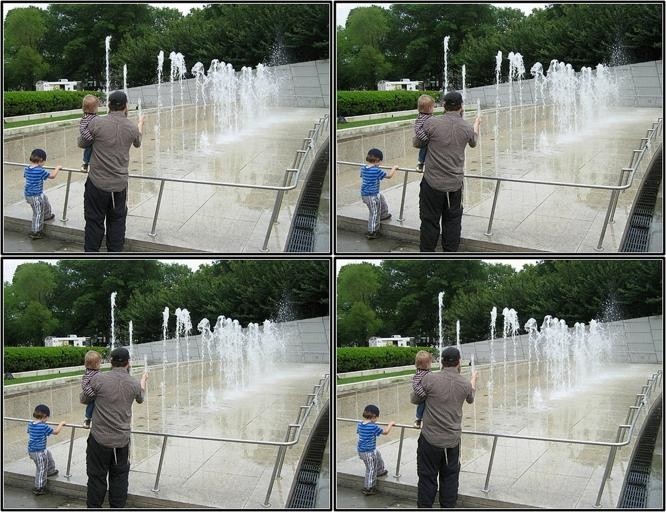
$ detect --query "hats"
[445,92,462,106]
[111,348,129,362]
[443,347,460,362]
[109,91,127,106]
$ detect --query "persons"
[75,90,144,253]
[409,347,479,508]
[413,92,481,252]
[358,148,398,239]
[22,148,62,239]
[82,350,103,429]
[78,349,148,508]
[25,404,64,496]
[413,95,435,173]
[78,94,100,170]
[355,405,395,496]
[413,350,432,429]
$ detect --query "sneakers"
[28,231,42,238]
[31,486,47,495]
[415,163,424,172]
[362,488,375,494]
[81,161,88,173]
[83,419,90,429]
[367,231,377,240]
[414,420,421,428]
